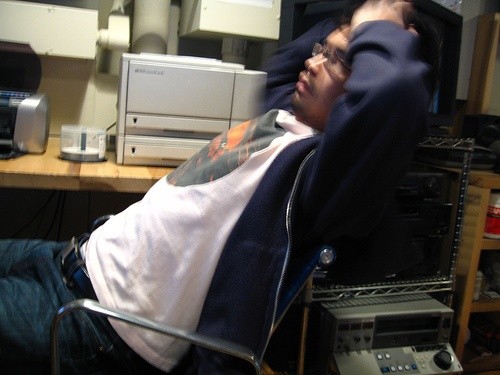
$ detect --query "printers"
[116,52,267,168]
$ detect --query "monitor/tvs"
[279,0,464,136]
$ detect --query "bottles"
[484,189,500,239]
[472,268,483,303]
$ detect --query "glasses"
[311,42,352,74]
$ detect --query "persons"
[0,0,437,375]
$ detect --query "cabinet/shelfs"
[440,169,500,375]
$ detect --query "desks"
[0,134,181,194]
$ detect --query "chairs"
[48,147,335,375]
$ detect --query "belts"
[61,232,108,306]
